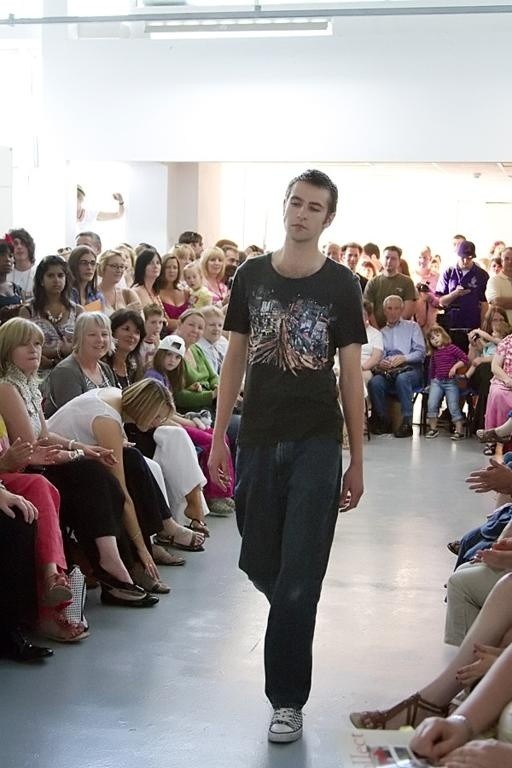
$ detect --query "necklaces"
[78,208,85,218]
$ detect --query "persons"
[0,228,270,661]
[323,233,512,767]
[207,170,369,741]
[76,184,126,247]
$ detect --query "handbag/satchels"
[371,350,413,388]
[61,565,89,631]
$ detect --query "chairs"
[423,328,477,437]
[387,355,427,435]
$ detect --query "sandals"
[31,607,91,643]
[92,513,210,607]
[447,540,460,555]
[475,429,512,456]
[43,571,73,608]
[349,692,449,729]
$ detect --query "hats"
[157,335,186,359]
[457,240,476,259]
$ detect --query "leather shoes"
[395,423,414,438]
[0,632,52,661]
[372,422,393,435]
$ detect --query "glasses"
[78,260,100,270]
[106,262,129,273]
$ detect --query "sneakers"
[207,496,235,514]
[456,373,470,385]
[450,431,466,440]
[426,428,440,438]
[268,706,302,743]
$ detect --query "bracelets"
[118,201,125,206]
